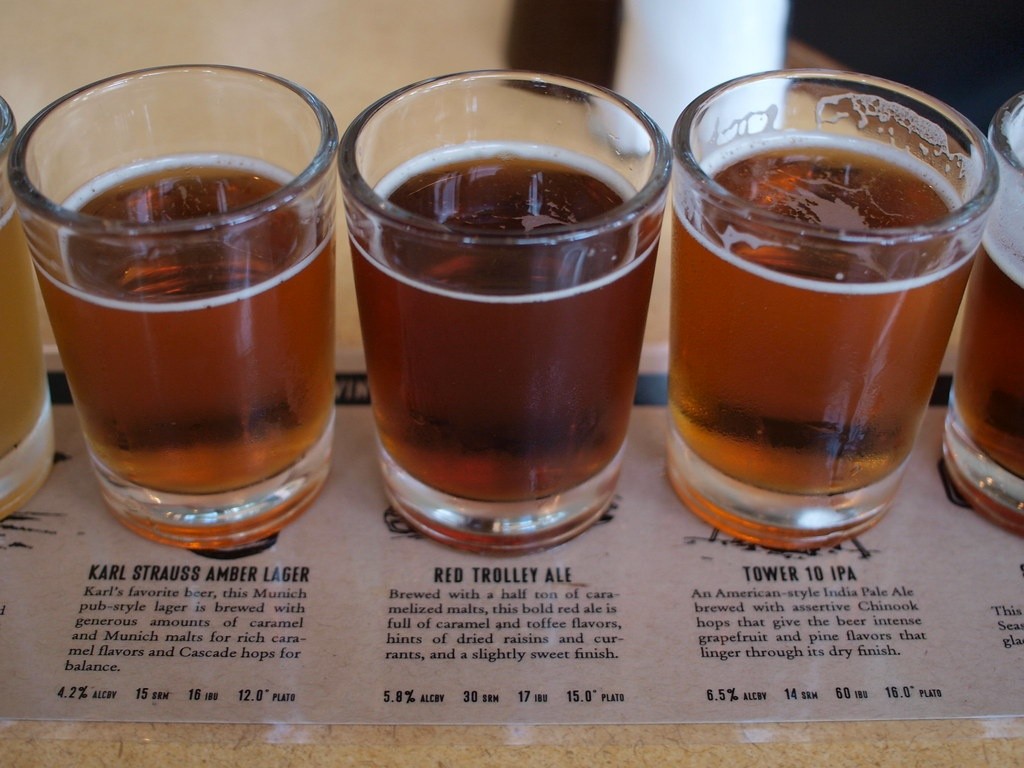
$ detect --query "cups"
[335,69,676,558]
[939,90,1024,537]
[5,63,340,549]
[664,68,999,551]
[0,96,57,524]
